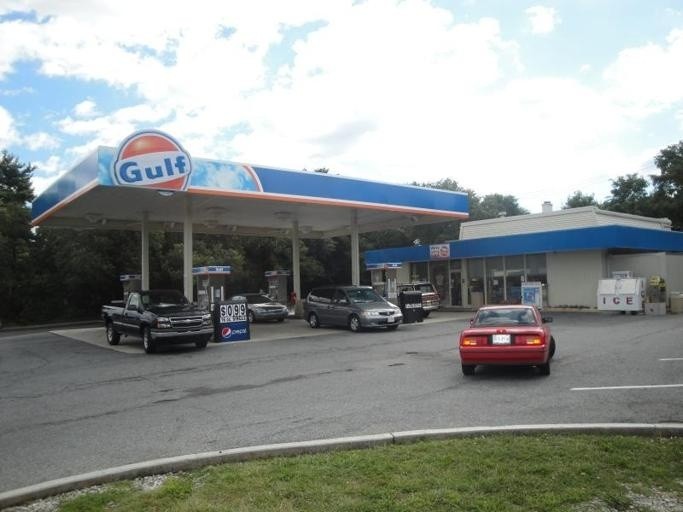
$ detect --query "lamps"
[206,207,227,217]
[157,190,174,197]
[204,220,218,228]
[300,225,312,234]
[84,214,102,224]
[274,213,289,226]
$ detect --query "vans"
[304,286,403,331]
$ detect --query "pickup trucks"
[101,290,214,354]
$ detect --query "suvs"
[400,282,440,316]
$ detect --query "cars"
[458,305,555,376]
[228,293,288,323]
